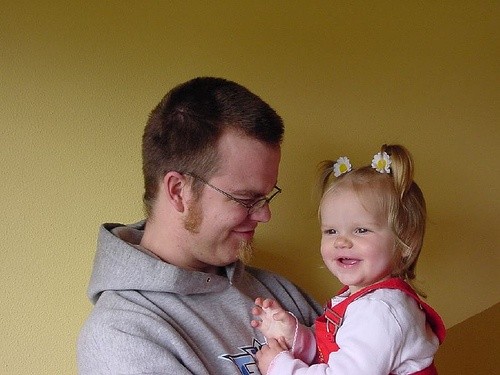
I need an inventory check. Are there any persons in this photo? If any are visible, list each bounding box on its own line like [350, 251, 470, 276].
[250, 142, 447, 375]
[74, 73, 325, 375]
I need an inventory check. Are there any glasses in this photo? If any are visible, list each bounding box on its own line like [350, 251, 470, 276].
[184, 173, 282, 213]
